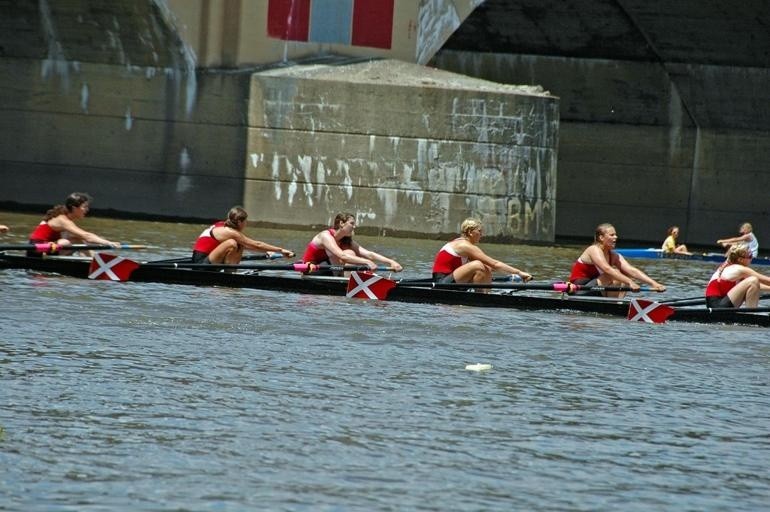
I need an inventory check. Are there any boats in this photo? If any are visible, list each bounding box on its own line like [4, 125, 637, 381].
[0, 253, 769, 326]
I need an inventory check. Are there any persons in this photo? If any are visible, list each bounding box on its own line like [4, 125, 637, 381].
[190, 205, 294, 273]
[0, 224, 9, 233]
[563, 222, 667, 303]
[661, 225, 692, 256]
[430, 215, 534, 293]
[25, 191, 121, 257]
[715, 222, 760, 259]
[300, 210, 404, 279]
[704, 241, 770, 314]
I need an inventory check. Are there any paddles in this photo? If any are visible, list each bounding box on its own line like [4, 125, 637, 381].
[88, 253, 396, 283]
[628, 300, 770, 322]
[347, 271, 666, 299]
